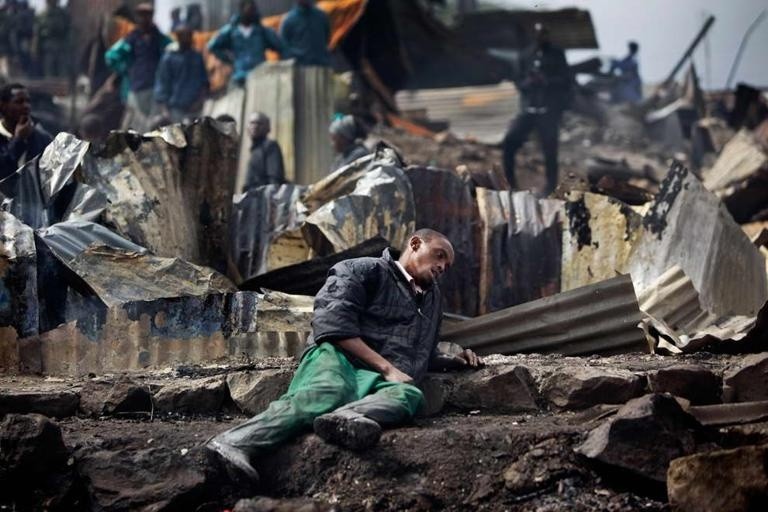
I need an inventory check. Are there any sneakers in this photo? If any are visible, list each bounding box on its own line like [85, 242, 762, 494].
[312, 404, 382, 455]
[202, 435, 260, 490]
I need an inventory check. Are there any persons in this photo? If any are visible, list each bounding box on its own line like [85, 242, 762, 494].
[81, 0, 330, 140]
[611, 40, 643, 102]
[0, 1, 68, 77]
[1, 83, 55, 178]
[500, 16, 583, 198]
[242, 112, 285, 191]
[203, 223, 490, 488]
[327, 114, 367, 173]
